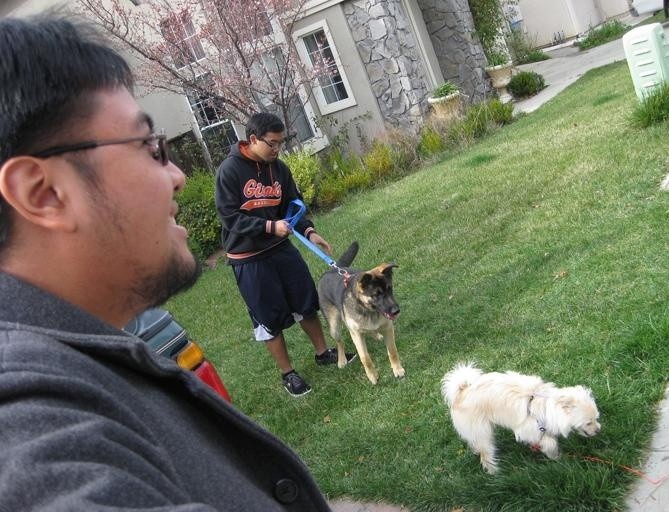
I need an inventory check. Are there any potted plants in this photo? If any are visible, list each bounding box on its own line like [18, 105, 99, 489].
[483, 49, 514, 104]
[426, 78, 464, 119]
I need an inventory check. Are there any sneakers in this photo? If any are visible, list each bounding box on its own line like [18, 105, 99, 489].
[282, 372, 312, 398]
[315, 348, 356, 367]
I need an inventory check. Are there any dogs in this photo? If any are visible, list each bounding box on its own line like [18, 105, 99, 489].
[317, 240, 406, 385]
[439, 361, 602, 477]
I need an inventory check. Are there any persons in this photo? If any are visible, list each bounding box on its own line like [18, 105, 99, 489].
[214, 112, 357, 397]
[0, 12, 337, 512]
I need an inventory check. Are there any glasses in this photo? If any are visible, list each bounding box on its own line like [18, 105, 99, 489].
[259, 136, 286, 150]
[31, 126, 169, 167]
